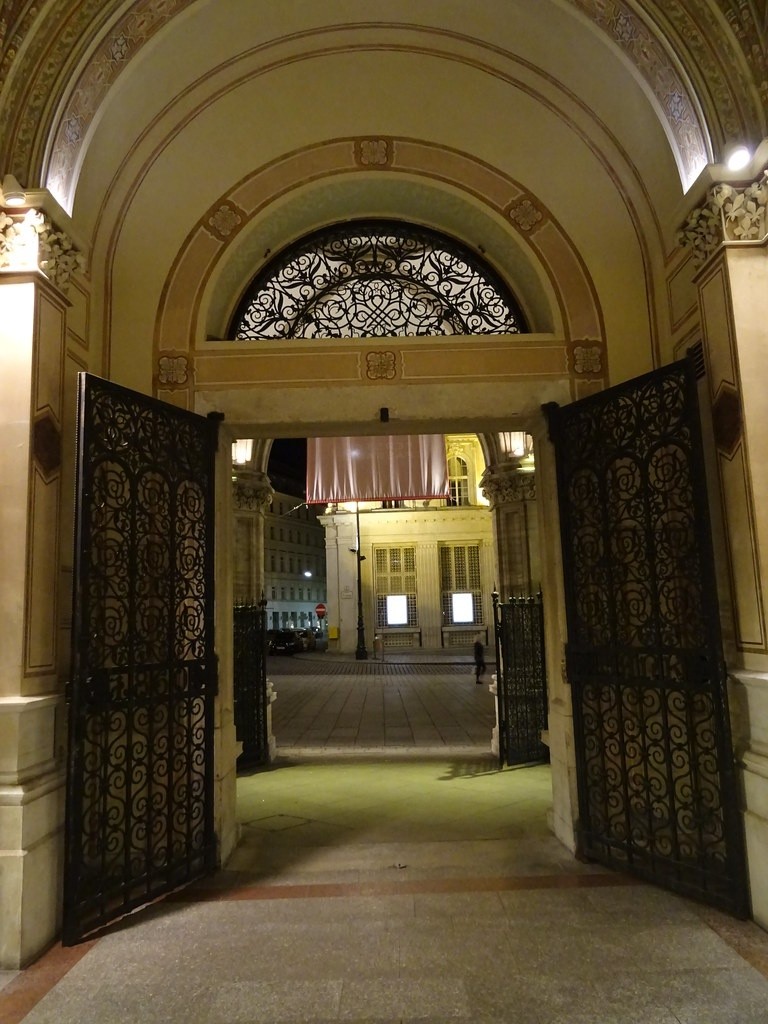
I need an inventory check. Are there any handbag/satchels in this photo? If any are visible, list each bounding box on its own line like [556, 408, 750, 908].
[473, 665, 478, 676]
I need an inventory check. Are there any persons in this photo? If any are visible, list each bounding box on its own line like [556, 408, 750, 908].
[301, 632, 308, 649]
[473, 634, 487, 684]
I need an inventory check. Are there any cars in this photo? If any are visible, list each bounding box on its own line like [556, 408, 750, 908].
[308, 627, 323, 639]
[269, 630, 316, 656]
[268, 626, 294, 646]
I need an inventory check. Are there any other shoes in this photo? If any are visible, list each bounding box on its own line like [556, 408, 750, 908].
[476, 680, 483, 684]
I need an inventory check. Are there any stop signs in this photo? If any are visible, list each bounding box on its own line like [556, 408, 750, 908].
[316, 604, 327, 618]
